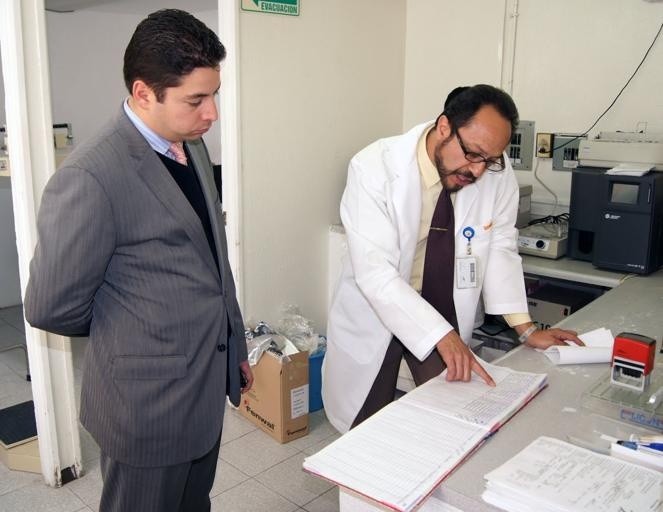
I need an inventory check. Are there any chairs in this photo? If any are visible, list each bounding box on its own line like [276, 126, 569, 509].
[395, 336, 484, 393]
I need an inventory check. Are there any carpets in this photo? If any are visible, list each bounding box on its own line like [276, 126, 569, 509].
[0, 399, 37, 449]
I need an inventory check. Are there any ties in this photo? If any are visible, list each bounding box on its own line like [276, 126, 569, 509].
[167, 140, 190, 168]
[420, 185, 456, 329]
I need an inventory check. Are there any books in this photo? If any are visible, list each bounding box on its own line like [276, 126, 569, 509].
[299, 345, 550, 512]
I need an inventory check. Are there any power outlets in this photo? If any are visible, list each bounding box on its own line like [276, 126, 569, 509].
[536, 133, 554, 158]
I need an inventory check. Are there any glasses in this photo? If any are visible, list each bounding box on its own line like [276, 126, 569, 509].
[449, 120, 507, 173]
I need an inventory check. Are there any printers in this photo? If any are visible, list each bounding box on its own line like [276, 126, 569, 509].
[576, 130, 663, 172]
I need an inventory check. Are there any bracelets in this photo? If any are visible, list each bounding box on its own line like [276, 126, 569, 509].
[517, 323, 537, 343]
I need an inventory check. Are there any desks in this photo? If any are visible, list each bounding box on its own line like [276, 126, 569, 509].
[339, 274, 663, 512]
[328, 225, 638, 364]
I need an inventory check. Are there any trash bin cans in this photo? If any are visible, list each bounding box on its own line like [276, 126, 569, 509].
[309, 335, 327, 412]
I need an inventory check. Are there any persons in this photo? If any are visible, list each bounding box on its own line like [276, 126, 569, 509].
[319, 83, 588, 434]
[22, 6, 258, 511]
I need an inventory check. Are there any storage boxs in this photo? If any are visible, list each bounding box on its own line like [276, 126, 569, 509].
[240, 324, 310, 445]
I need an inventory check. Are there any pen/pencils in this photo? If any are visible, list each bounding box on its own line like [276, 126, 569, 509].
[617, 440, 663, 451]
